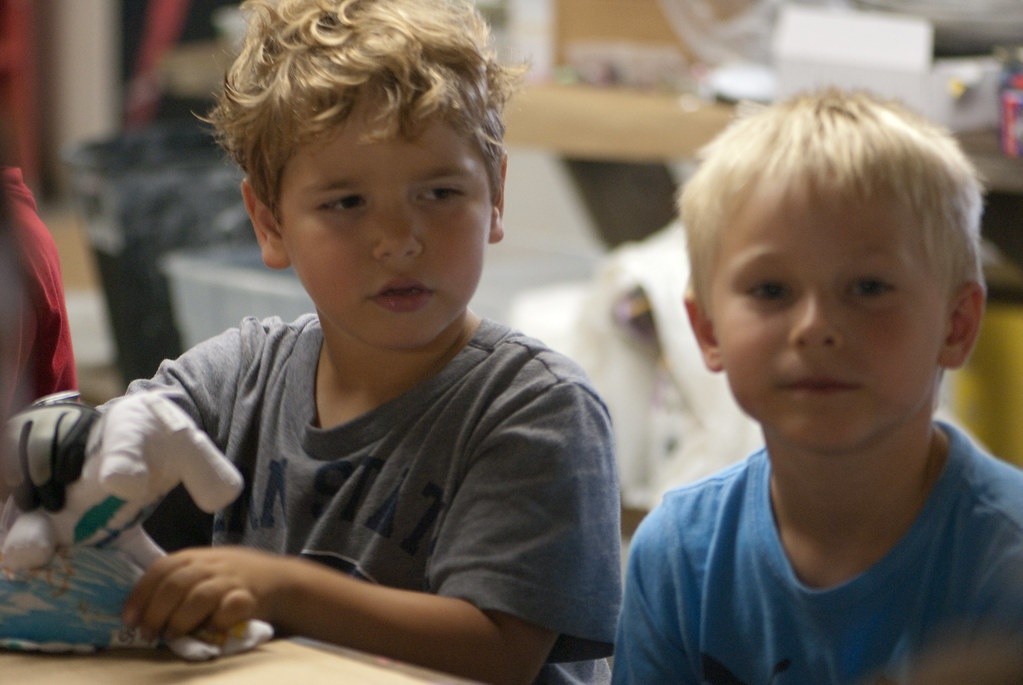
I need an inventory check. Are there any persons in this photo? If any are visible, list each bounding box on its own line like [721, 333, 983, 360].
[611, 84, 1023, 685]
[0, 65, 81, 504]
[3, 0, 625, 685]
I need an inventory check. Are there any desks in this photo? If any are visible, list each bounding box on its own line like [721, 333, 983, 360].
[0, 597, 482, 684]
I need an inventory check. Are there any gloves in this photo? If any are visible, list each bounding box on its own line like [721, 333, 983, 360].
[2, 388, 103, 511]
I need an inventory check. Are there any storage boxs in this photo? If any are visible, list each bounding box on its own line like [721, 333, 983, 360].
[165, 243, 314, 354]
